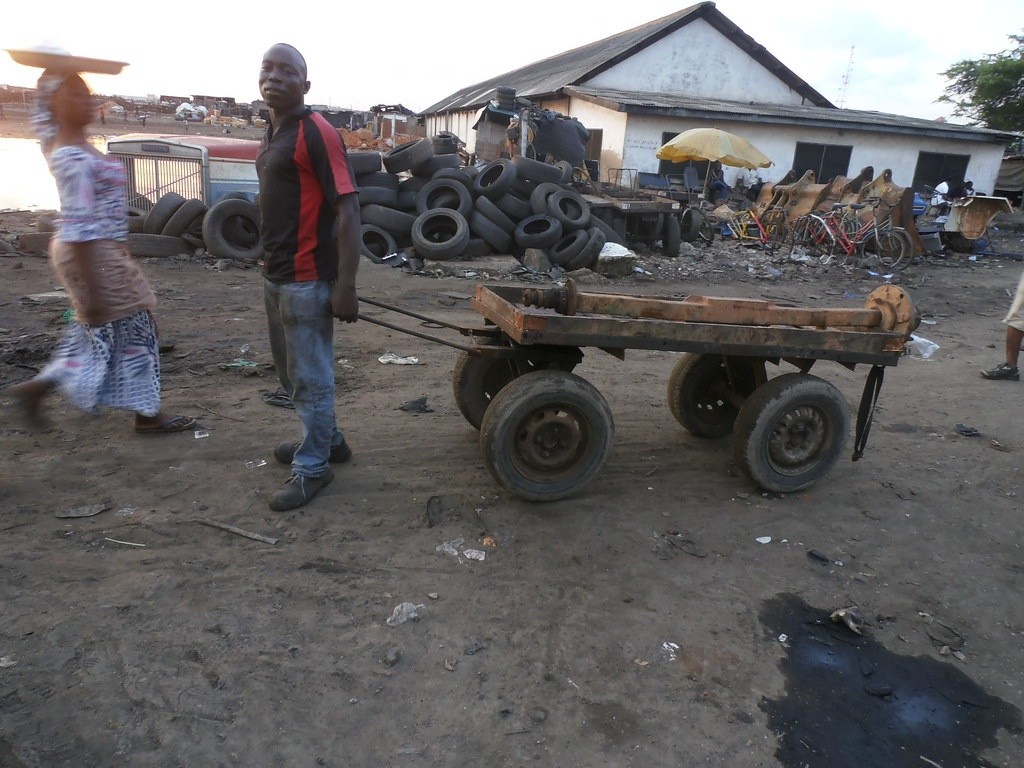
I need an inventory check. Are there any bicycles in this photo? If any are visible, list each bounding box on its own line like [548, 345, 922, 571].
[724, 194, 916, 275]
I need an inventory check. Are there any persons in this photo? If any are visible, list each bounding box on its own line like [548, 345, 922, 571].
[977, 259, 1024, 383]
[121, 107, 129, 125]
[0, 100, 8, 120]
[140, 116, 146, 129]
[248, 40, 364, 516]
[734, 162, 770, 196]
[705, 159, 734, 195]
[6, 43, 199, 438]
[99, 106, 106, 125]
[134, 104, 140, 118]
[182, 116, 189, 130]
[931, 176, 954, 214]
[953, 179, 975, 198]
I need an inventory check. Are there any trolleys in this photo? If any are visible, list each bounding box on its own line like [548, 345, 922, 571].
[356, 279, 912, 507]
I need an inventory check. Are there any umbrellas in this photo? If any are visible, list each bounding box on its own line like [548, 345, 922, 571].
[655, 119, 774, 198]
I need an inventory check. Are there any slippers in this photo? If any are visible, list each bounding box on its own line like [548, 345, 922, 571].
[134, 412, 198, 433]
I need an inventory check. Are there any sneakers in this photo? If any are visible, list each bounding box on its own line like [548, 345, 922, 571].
[980, 360, 1020, 382]
[269, 465, 335, 512]
[273, 438, 353, 467]
